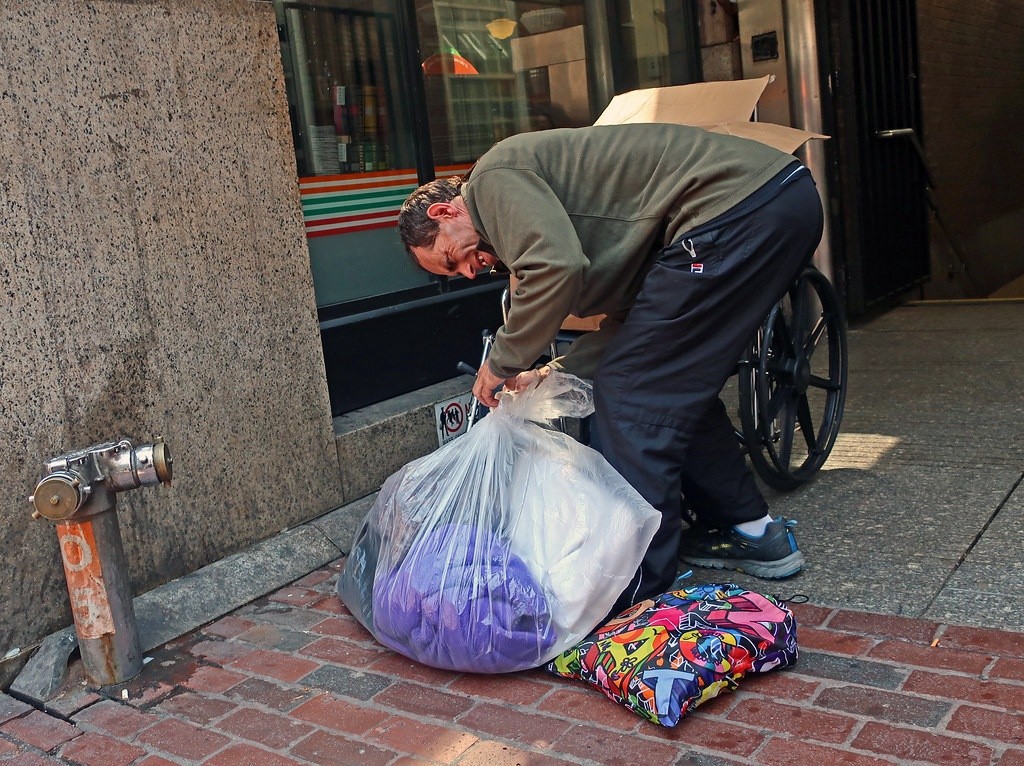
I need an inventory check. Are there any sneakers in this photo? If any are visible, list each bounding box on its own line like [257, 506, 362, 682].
[679, 518, 805, 580]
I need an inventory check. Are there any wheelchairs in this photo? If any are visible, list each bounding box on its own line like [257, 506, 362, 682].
[455, 261, 848, 491]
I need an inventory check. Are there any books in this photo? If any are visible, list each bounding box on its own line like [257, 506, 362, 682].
[296, 4, 410, 170]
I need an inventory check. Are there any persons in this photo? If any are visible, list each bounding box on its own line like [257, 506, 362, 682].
[397, 122, 824, 618]
